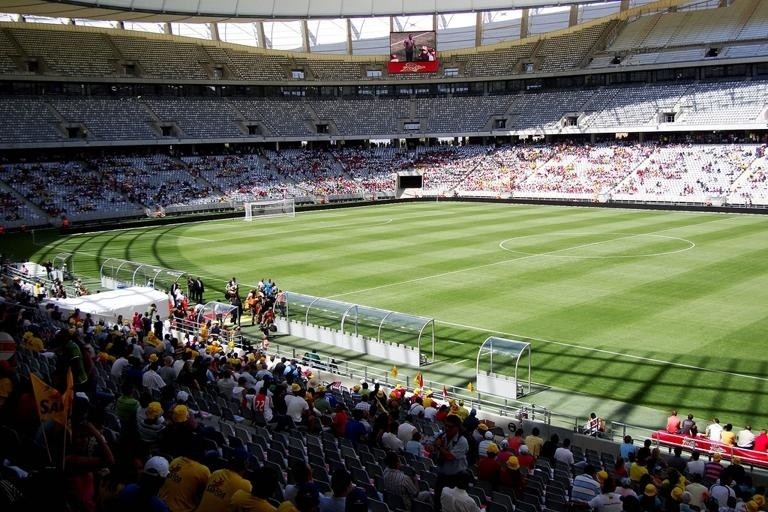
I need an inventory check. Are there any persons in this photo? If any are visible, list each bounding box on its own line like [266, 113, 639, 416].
[0, 255, 288, 341]
[0, 314, 768, 512]
[403, 33, 418, 64]
[478, 138, 768, 208]
[0, 141, 480, 229]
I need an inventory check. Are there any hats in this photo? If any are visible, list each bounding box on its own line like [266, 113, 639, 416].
[595, 470, 609, 483]
[671, 487, 691, 502]
[488, 438, 529, 471]
[145, 402, 165, 420]
[177, 390, 189, 402]
[148, 353, 173, 365]
[144, 456, 171, 479]
[173, 404, 189, 422]
[477, 423, 493, 440]
[74, 391, 90, 410]
[294, 482, 321, 507]
[644, 484, 658, 497]
[746, 495, 765, 510]
[229, 449, 253, 467]
[345, 486, 368, 511]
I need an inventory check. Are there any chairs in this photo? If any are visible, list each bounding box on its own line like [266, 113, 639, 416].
[0, 317, 768, 512]
